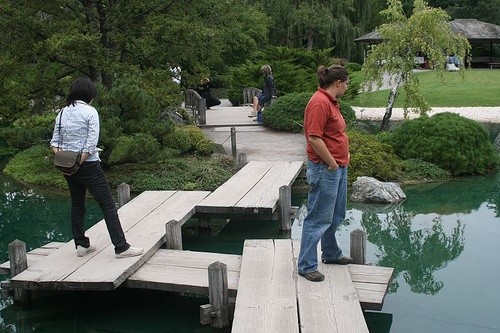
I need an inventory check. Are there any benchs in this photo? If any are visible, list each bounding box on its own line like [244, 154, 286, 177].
[488, 63, 500, 69]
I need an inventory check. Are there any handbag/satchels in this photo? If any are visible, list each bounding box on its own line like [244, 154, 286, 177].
[54, 151, 80, 173]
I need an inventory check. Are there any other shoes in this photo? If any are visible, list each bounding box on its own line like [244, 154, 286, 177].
[253, 118, 258, 121]
[248, 112, 257, 117]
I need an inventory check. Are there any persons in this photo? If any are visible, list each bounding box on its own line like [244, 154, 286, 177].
[195, 79, 221, 109]
[297, 64, 353, 282]
[50, 78, 143, 258]
[248, 64, 277, 121]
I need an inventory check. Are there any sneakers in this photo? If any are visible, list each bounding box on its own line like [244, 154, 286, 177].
[322, 256, 353, 265]
[298, 271, 324, 282]
[116, 247, 144, 259]
[75, 244, 95, 257]
